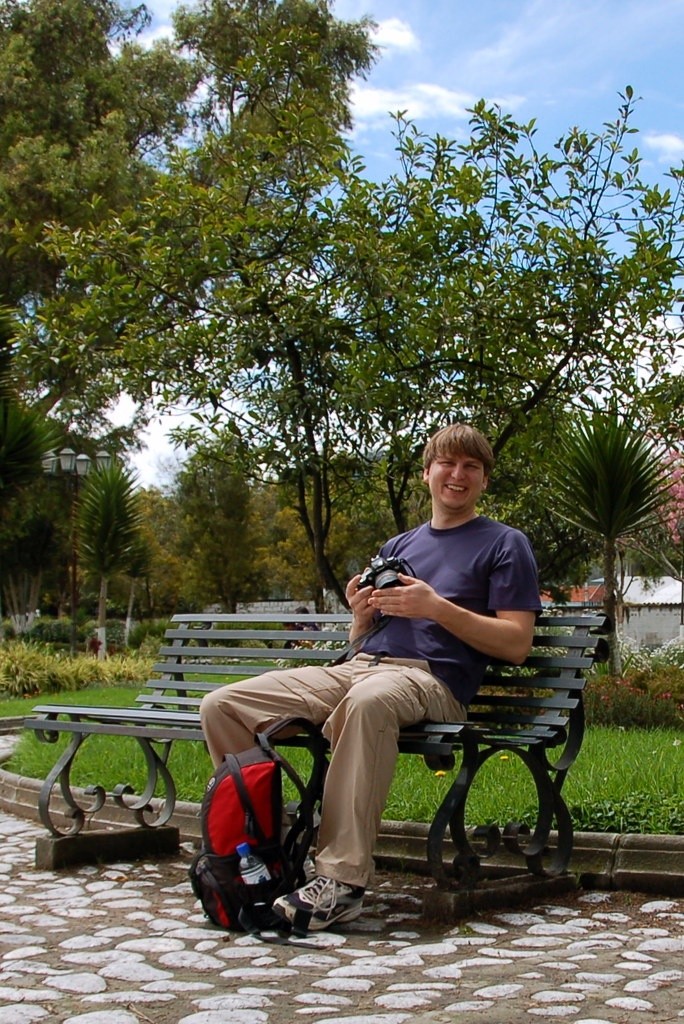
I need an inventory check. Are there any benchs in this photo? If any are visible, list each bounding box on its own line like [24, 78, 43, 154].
[24, 612, 614, 892]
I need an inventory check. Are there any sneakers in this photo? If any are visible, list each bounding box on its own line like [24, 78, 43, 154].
[272, 877, 364, 929]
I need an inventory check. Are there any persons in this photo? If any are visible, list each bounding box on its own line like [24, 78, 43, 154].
[199, 425, 542, 933]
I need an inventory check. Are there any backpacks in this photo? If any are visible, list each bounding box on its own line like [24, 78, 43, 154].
[189, 718, 328, 938]
